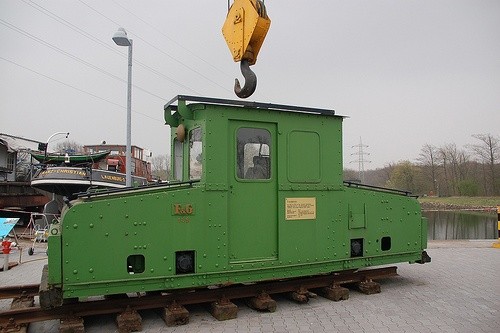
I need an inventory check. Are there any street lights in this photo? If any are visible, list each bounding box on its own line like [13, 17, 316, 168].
[112, 25, 134, 190]
[46, 133, 69, 146]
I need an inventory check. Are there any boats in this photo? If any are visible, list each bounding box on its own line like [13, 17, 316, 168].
[32, 143, 156, 200]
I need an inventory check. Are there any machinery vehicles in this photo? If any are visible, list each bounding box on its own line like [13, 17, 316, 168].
[34, 96, 432, 301]
